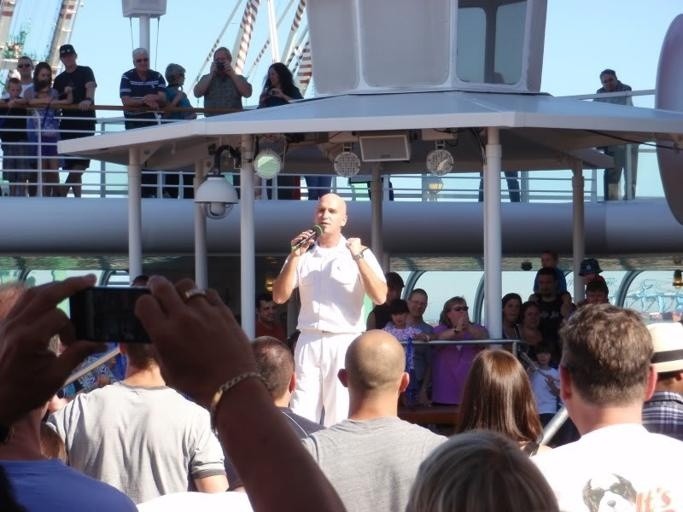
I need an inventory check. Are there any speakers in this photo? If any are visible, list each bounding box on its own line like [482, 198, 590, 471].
[359, 129, 411, 162]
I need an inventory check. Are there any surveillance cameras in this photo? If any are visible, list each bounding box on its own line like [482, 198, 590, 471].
[521, 264, 532, 270]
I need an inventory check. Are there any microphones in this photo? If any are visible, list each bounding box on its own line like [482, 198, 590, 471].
[291, 224, 323, 250]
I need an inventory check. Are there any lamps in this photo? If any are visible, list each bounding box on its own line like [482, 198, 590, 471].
[248, 130, 461, 183]
[192, 144, 245, 222]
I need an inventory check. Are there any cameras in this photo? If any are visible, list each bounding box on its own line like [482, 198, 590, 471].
[216, 63, 224, 69]
[269, 90, 276, 96]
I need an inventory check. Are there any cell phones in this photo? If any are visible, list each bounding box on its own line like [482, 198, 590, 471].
[70, 286, 154, 342]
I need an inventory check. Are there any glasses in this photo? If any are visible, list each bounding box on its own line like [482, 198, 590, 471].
[602, 77, 616, 84]
[16, 63, 33, 69]
[448, 306, 469, 312]
[134, 58, 150, 63]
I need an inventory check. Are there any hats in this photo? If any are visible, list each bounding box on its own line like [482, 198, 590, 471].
[642, 320, 683, 376]
[577, 258, 604, 277]
[59, 44, 75, 59]
